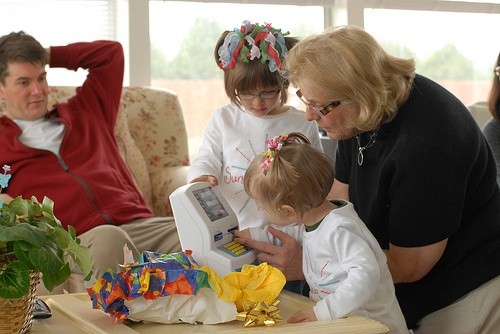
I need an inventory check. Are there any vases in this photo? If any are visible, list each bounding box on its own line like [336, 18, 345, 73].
[0, 267, 39, 334]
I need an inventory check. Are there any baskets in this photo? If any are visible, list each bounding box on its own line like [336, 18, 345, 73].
[0, 267, 40, 334]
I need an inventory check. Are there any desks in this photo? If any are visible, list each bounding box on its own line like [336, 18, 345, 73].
[31, 290, 389, 334]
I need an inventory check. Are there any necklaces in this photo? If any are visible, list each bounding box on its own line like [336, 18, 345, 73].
[356, 129, 377, 166]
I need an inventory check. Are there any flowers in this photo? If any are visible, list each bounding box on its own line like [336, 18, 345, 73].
[0, 163, 93, 298]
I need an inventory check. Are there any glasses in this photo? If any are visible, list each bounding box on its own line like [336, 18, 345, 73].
[235, 87, 280, 99]
[296, 89, 351, 118]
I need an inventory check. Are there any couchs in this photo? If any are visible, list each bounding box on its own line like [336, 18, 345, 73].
[0, 86, 192, 216]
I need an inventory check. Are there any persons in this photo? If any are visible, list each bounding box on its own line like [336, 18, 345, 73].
[482, 53, 500, 187]
[232, 132, 409, 334]
[233, 24, 500, 334]
[189, 20, 339, 231]
[0, 32, 182, 291]
